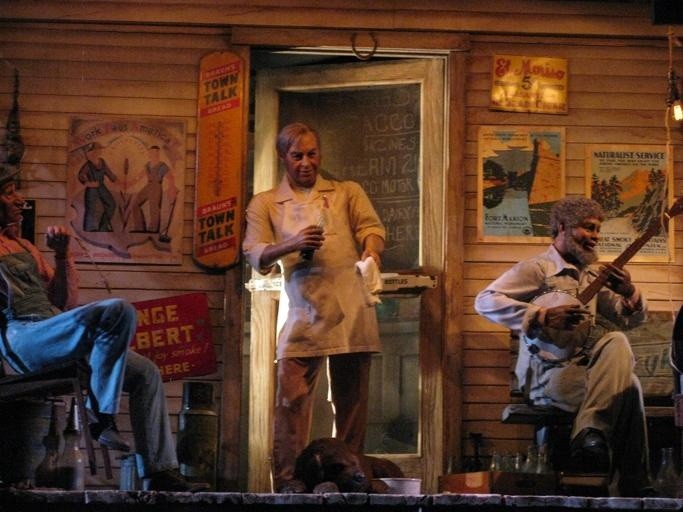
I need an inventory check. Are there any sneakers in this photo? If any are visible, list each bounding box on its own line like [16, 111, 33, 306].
[143, 468, 212, 491]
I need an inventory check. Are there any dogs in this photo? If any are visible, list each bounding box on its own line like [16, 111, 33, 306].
[278, 438, 406, 494]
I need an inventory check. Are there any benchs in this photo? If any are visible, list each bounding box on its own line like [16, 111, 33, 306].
[501, 309, 683, 477]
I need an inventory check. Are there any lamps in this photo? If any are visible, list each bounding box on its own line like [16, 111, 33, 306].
[665, 69, 683, 122]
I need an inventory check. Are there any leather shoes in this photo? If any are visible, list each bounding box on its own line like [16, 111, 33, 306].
[83, 407, 131, 452]
[571, 428, 609, 466]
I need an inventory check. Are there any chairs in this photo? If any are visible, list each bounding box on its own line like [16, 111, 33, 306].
[0, 371, 115, 477]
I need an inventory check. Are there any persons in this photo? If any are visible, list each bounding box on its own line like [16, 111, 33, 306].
[80, 141, 123, 234]
[239, 120, 389, 496]
[123, 146, 178, 234]
[473, 195, 660, 497]
[1, 162, 210, 492]
